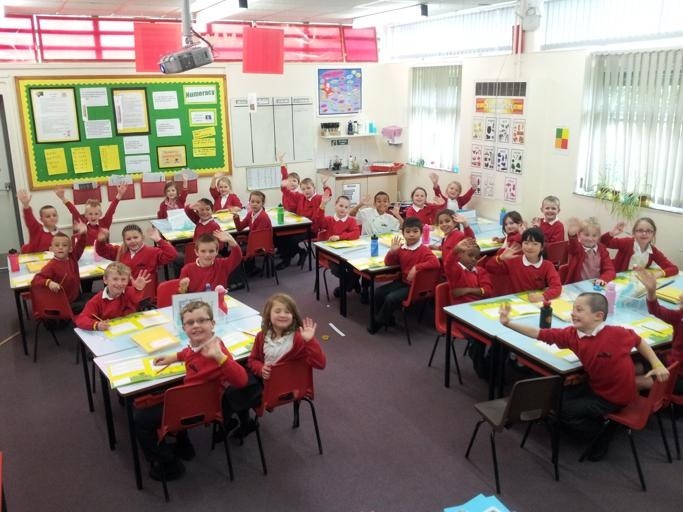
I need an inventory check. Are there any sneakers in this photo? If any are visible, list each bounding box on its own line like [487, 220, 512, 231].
[229, 420, 259, 441]
[150, 441, 195, 480]
[333, 272, 369, 304]
[276, 260, 290, 270]
[297, 248, 307, 265]
[216, 418, 238, 442]
[368, 316, 395, 334]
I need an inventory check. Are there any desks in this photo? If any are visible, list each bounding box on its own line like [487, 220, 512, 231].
[93, 314, 268, 490]
[616, 262, 682, 304]
[443, 277, 645, 406]
[73, 292, 261, 414]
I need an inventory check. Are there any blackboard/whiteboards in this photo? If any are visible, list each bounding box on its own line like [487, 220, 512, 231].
[230, 96, 315, 168]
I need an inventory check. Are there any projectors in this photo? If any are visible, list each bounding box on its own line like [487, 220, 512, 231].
[159, 45, 213, 74]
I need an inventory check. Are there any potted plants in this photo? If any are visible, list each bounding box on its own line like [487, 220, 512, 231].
[594, 170, 652, 225]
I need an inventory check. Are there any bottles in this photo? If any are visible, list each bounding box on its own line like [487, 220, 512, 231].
[214, 284, 228, 314]
[348, 154, 353, 169]
[538, 297, 553, 330]
[421, 223, 429, 245]
[593, 285, 601, 294]
[370, 234, 378, 256]
[276, 203, 283, 224]
[93, 240, 102, 262]
[499, 207, 506, 225]
[604, 281, 615, 312]
[347, 120, 361, 136]
[7, 248, 20, 272]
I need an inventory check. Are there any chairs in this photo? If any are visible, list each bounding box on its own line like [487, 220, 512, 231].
[425, 274, 494, 381]
[574, 362, 682, 491]
[138, 372, 236, 501]
[7, 204, 562, 362]
[211, 352, 324, 475]
[464, 373, 564, 495]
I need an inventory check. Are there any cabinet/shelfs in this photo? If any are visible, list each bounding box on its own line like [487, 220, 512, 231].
[316, 161, 398, 218]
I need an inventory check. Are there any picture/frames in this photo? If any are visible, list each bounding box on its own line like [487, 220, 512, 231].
[317, 68, 363, 115]
[28, 86, 81, 143]
[111, 87, 150, 136]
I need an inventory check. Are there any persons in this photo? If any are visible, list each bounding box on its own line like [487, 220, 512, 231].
[564, 215, 617, 284]
[486, 195, 562, 301]
[18, 189, 58, 254]
[221, 291, 326, 438]
[443, 236, 508, 383]
[134, 300, 249, 482]
[276, 151, 332, 271]
[157, 174, 273, 289]
[600, 218, 677, 278]
[30, 217, 178, 347]
[54, 183, 128, 246]
[312, 172, 479, 334]
[633, 263, 683, 396]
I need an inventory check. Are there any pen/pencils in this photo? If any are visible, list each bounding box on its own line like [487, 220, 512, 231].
[642, 325, 664, 334]
[320, 122, 339, 132]
[157, 364, 170, 374]
[242, 332, 256, 337]
[59, 273, 67, 287]
[91, 313, 112, 326]
